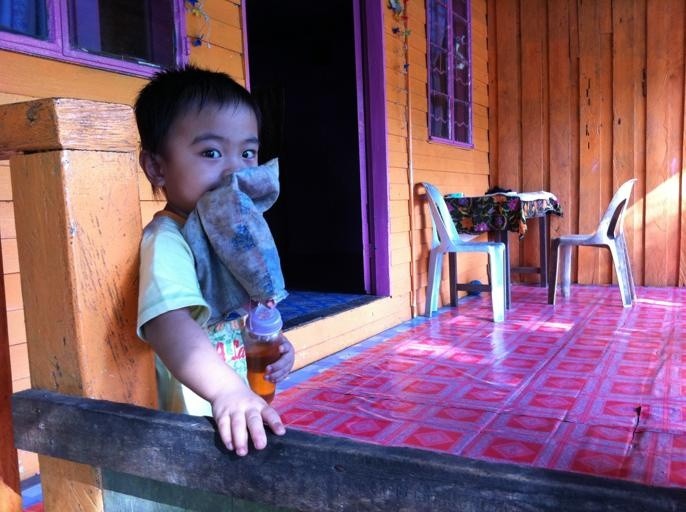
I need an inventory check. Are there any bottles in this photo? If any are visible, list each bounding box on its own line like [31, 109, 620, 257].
[242, 296, 283, 404]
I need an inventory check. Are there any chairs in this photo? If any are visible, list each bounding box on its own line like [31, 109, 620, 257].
[547, 178, 639, 308]
[421, 181, 506, 321]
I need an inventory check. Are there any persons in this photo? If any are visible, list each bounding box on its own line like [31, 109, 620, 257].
[133, 61, 296, 458]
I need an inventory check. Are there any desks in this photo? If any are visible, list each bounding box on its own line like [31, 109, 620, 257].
[440, 190, 564, 308]
